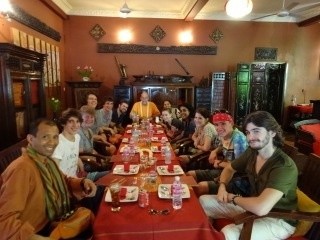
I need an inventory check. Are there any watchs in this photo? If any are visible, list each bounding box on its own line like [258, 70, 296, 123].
[80, 178, 85, 189]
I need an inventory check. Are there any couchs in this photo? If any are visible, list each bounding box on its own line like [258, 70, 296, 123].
[293, 120, 320, 156]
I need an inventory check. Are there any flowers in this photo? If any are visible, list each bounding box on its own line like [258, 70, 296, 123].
[75, 64, 93, 78]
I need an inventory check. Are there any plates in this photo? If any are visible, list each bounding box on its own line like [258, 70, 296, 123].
[152, 146, 166, 152]
[127, 130, 132, 134]
[155, 165, 185, 175]
[156, 124, 162, 128]
[151, 137, 168, 141]
[298, 103, 309, 106]
[120, 147, 139, 153]
[122, 137, 129, 143]
[105, 186, 139, 203]
[127, 124, 132, 127]
[157, 130, 164, 133]
[113, 165, 139, 175]
[158, 184, 191, 199]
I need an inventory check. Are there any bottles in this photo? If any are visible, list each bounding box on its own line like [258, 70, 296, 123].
[130, 145, 135, 164]
[148, 157, 157, 179]
[139, 155, 148, 179]
[139, 188, 145, 207]
[123, 145, 129, 163]
[172, 176, 182, 210]
[165, 142, 172, 164]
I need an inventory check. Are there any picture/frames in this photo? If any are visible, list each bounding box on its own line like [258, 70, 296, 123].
[253, 46, 278, 62]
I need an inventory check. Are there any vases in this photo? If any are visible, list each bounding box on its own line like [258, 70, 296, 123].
[82, 77, 89, 82]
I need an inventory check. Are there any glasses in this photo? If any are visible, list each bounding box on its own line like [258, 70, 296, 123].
[213, 109, 232, 115]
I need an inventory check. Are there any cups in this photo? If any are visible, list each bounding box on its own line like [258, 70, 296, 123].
[168, 163, 173, 171]
[158, 143, 161, 151]
[291, 96, 296, 106]
[124, 161, 129, 170]
[109, 182, 121, 212]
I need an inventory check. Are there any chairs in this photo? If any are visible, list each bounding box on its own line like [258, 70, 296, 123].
[176, 136, 191, 145]
[215, 143, 320, 240]
[79, 139, 110, 163]
[97, 127, 113, 142]
[196, 153, 216, 169]
[81, 158, 101, 172]
[0, 138, 26, 174]
[180, 140, 210, 162]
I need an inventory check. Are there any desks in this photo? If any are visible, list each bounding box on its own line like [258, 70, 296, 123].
[287, 103, 314, 121]
[309, 99, 320, 119]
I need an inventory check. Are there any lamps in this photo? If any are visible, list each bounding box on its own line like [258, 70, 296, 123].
[1, 1, 15, 23]
[224, 0, 253, 19]
[118, 0, 131, 18]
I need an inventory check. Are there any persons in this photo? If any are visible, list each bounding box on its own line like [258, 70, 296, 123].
[52, 108, 109, 195]
[95, 98, 122, 143]
[187, 110, 250, 193]
[168, 103, 196, 143]
[0, 118, 94, 239]
[79, 105, 113, 170]
[175, 106, 220, 167]
[199, 111, 299, 240]
[111, 101, 129, 127]
[129, 91, 161, 121]
[83, 92, 120, 154]
[160, 100, 182, 128]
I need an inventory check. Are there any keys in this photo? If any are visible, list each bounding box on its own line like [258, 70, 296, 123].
[148, 207, 170, 216]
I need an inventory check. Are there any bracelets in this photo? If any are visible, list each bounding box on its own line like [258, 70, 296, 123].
[219, 182, 226, 186]
[217, 160, 223, 168]
[231, 194, 242, 206]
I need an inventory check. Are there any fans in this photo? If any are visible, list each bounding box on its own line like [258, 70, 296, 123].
[250, 0, 305, 23]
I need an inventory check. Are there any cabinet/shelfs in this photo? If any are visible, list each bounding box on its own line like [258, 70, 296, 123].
[209, 72, 230, 114]
[234, 63, 251, 126]
[195, 86, 210, 118]
[250, 63, 265, 113]
[264, 62, 289, 123]
[132, 82, 194, 116]
[112, 85, 133, 124]
[65, 80, 104, 109]
[0, 42, 49, 151]
